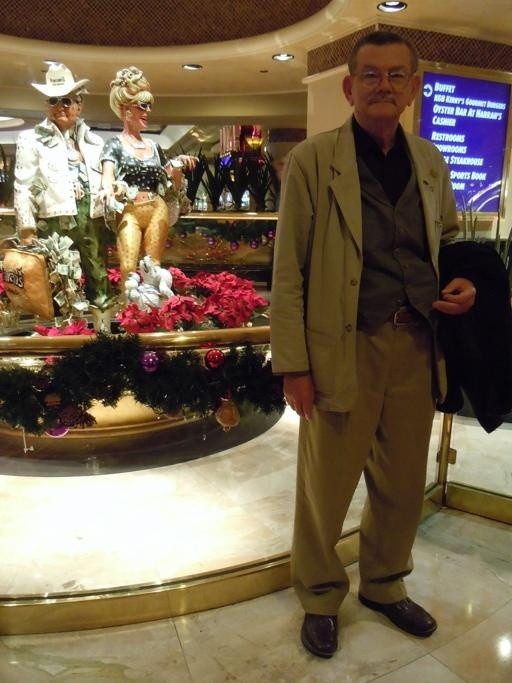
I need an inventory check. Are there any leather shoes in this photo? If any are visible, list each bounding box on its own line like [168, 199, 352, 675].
[301, 613, 337, 658]
[359, 592, 437, 636]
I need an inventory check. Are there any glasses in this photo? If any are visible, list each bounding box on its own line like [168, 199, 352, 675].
[49, 97, 72, 108]
[133, 103, 152, 112]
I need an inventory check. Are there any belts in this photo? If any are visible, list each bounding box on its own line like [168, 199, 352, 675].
[386, 310, 424, 326]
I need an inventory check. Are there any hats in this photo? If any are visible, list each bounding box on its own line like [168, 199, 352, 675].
[31, 62, 90, 97]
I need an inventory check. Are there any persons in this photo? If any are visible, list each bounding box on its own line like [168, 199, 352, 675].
[100, 68, 197, 295]
[15, 64, 104, 307]
[269, 31, 478, 659]
